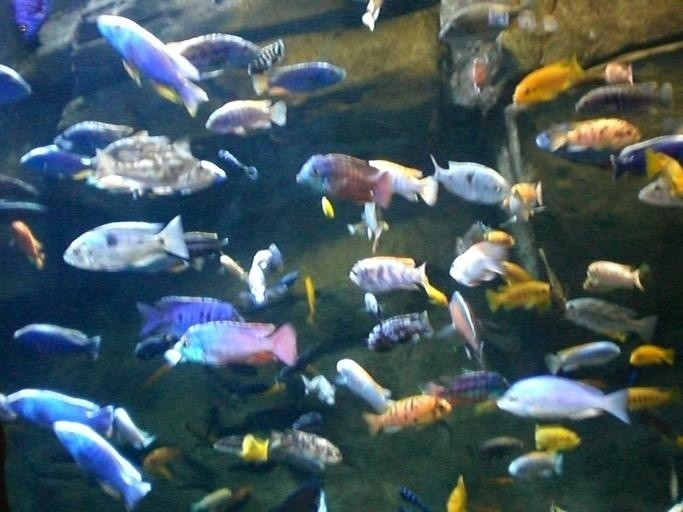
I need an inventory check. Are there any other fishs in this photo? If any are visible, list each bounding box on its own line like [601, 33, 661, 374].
[0, 1, 682, 511]
